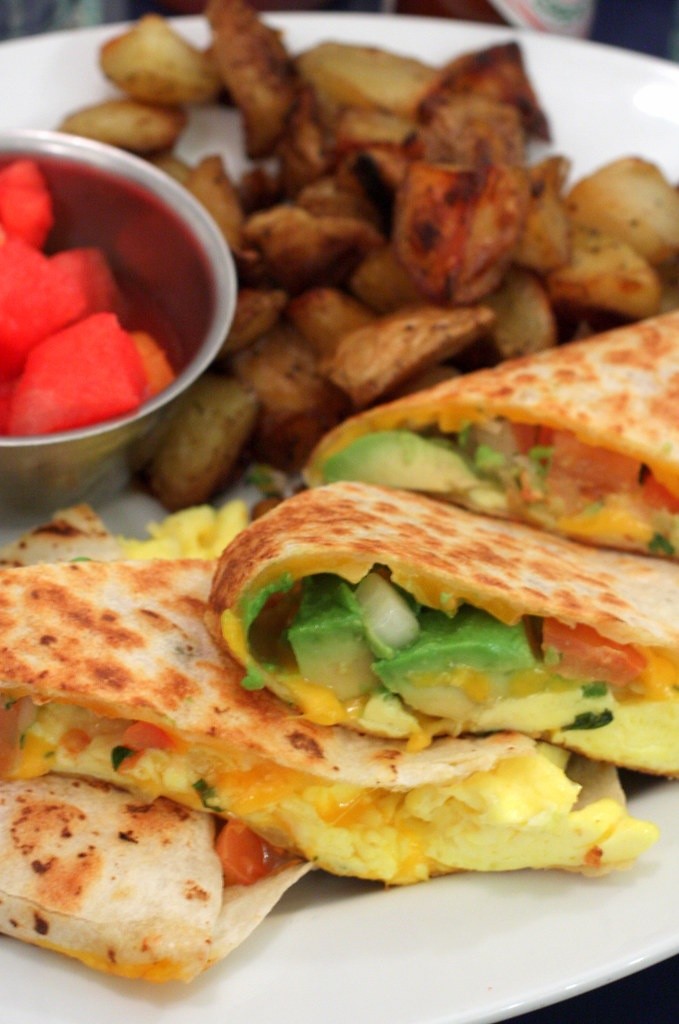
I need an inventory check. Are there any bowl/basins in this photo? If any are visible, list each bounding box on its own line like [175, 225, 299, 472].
[0, 127, 238, 526]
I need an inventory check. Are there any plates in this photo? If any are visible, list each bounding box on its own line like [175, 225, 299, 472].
[0, 13, 679, 1024]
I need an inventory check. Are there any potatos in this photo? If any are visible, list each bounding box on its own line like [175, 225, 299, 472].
[57, 0, 679, 514]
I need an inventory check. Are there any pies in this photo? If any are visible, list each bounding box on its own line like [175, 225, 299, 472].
[0, 308, 679, 987]
